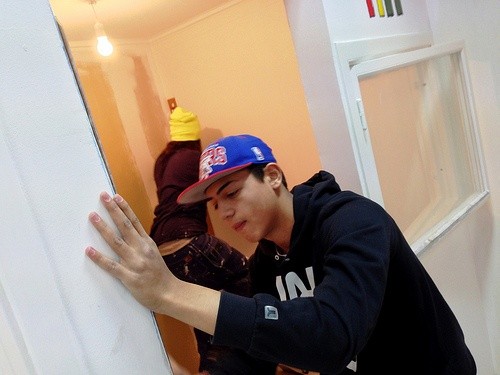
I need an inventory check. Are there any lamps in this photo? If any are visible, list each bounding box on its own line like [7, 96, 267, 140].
[93, 22, 114, 57]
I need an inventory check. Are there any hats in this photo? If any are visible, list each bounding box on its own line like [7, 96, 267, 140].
[177, 135, 277, 204]
[169, 107, 201, 141]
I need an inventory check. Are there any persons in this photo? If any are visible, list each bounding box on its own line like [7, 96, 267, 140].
[86, 135, 477, 375]
[149, 107, 249, 374]
